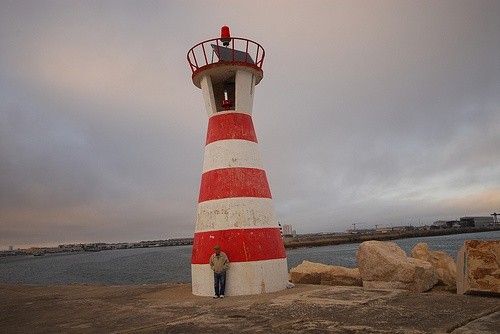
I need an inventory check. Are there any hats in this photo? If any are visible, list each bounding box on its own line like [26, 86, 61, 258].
[214, 245, 221, 249]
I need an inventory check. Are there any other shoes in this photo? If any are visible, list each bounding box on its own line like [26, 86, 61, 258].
[219, 295, 224, 298]
[213, 295, 218, 299]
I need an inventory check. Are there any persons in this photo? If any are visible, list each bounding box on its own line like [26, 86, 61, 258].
[209, 245, 229, 299]
